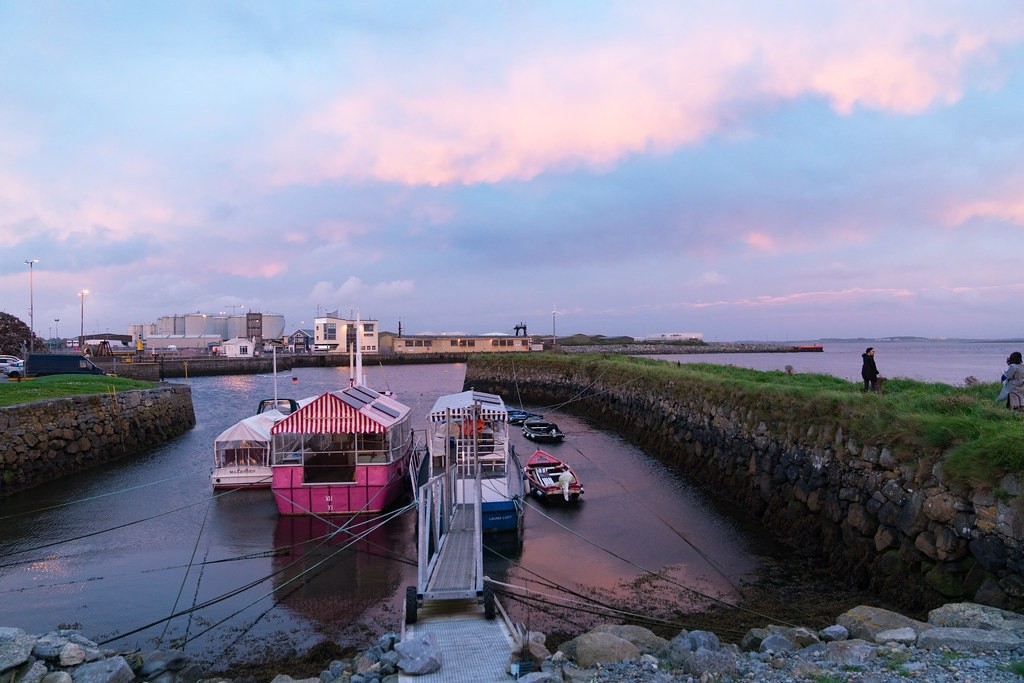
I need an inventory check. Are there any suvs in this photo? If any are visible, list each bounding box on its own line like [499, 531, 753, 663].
[0, 355, 21, 373]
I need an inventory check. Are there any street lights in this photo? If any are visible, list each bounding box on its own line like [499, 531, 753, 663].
[78, 290, 89, 348]
[23, 259, 38, 353]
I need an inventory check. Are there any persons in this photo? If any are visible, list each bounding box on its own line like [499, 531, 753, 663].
[460, 417, 486, 444]
[996, 351, 1024, 412]
[861, 347, 879, 393]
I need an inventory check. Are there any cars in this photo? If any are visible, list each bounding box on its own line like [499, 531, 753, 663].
[2, 360, 26, 378]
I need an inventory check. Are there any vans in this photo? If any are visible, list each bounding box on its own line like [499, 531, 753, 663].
[27, 353, 104, 378]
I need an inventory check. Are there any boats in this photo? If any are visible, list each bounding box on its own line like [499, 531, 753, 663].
[521, 416, 566, 444]
[504, 405, 545, 426]
[409, 388, 526, 546]
[208, 393, 324, 488]
[269, 312, 418, 517]
[524, 446, 584, 506]
